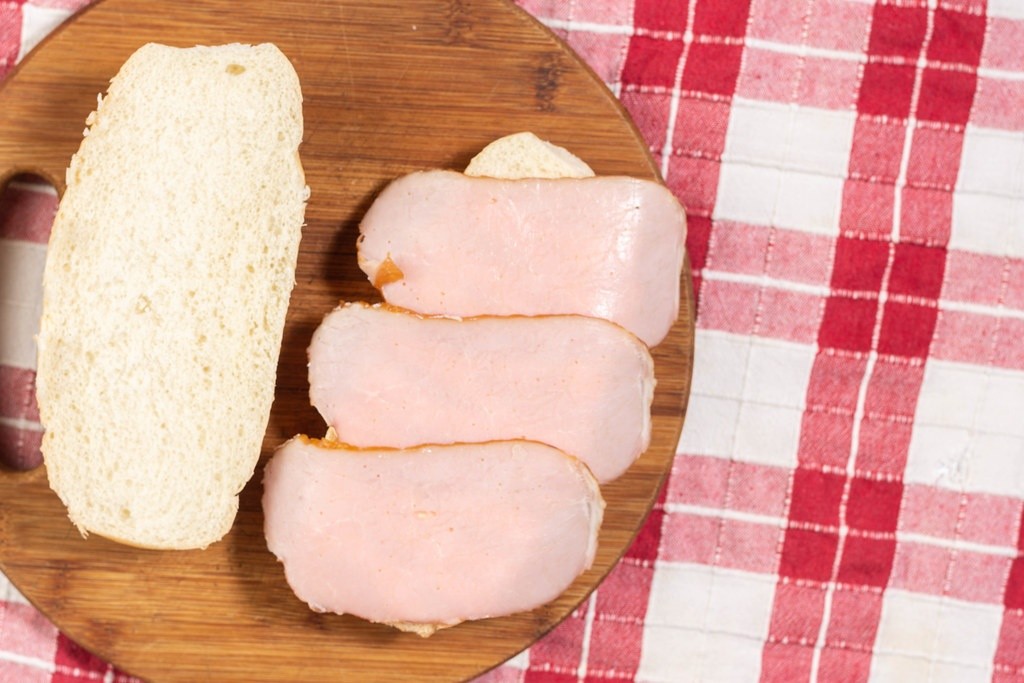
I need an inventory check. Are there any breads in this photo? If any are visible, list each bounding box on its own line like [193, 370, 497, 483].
[325, 133, 595, 637]
[38, 42, 311, 551]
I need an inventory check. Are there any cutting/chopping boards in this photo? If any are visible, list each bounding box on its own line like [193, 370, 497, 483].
[0, 1, 694, 683]
